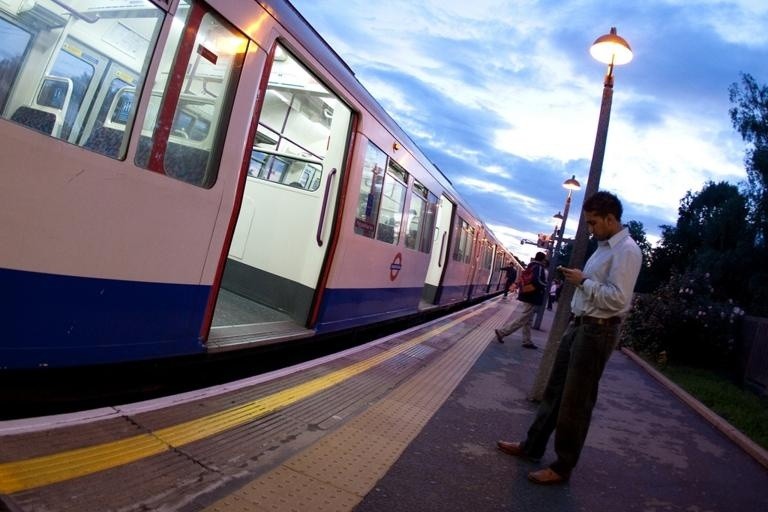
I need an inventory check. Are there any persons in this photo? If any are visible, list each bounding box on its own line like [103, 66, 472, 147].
[499, 261, 517, 300]
[530, 257, 551, 330]
[546, 279, 558, 311]
[492, 190, 644, 485]
[494, 251, 548, 349]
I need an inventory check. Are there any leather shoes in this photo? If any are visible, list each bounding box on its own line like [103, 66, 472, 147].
[527, 467, 564, 484]
[496, 439, 541, 463]
[495, 329, 504, 343]
[521, 343, 538, 349]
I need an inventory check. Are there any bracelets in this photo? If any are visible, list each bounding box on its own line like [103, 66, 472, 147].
[578, 276, 590, 289]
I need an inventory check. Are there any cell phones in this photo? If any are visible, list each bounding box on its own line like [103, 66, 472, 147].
[556, 266, 568, 274]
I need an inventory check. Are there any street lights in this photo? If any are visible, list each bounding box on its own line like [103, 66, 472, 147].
[530, 27, 634, 406]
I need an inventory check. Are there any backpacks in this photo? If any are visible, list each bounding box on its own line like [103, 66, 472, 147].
[517, 263, 538, 293]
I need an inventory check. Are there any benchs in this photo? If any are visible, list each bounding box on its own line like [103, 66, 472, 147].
[9, 105, 209, 189]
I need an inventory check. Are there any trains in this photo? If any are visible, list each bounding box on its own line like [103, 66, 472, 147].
[0, 0, 526, 370]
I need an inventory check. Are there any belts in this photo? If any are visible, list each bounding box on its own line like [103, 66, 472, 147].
[568, 313, 621, 326]
